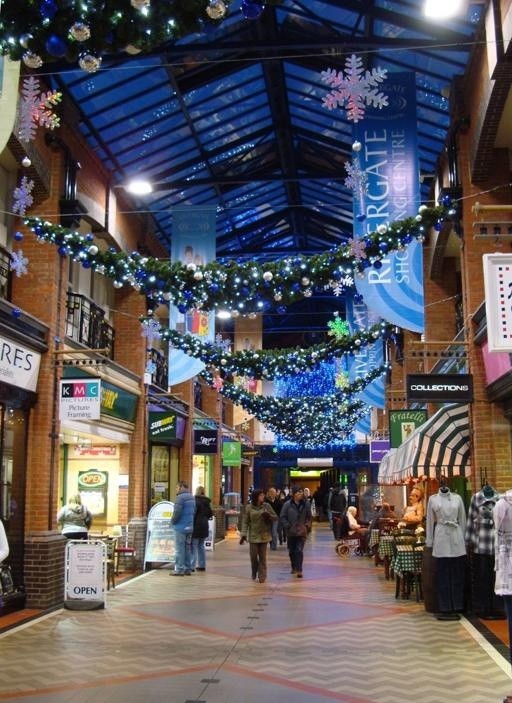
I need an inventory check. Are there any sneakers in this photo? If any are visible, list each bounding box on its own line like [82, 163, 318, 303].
[292, 569, 303, 578]
[169, 567, 205, 575]
[252, 571, 265, 582]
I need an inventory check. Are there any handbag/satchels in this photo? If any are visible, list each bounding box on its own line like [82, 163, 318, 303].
[84, 516, 91, 525]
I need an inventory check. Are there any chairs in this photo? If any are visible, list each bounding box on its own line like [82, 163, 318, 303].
[378, 521, 426, 603]
[89, 535, 109, 544]
[100, 539, 116, 591]
[114, 531, 137, 576]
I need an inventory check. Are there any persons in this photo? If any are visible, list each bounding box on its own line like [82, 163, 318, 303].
[190, 485, 212, 572]
[493, 487, 512, 662]
[0, 520, 10, 562]
[401, 487, 425, 529]
[169, 480, 196, 577]
[57, 492, 92, 544]
[425, 489, 465, 613]
[238, 483, 347, 583]
[182, 245, 193, 266]
[193, 254, 201, 265]
[367, 502, 398, 539]
[345, 506, 369, 552]
[467, 484, 499, 618]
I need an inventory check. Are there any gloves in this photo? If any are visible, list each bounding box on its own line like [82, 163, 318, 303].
[240, 536, 247, 544]
[260, 512, 271, 520]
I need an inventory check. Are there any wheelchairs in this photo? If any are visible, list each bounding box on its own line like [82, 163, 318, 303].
[332, 513, 373, 557]
